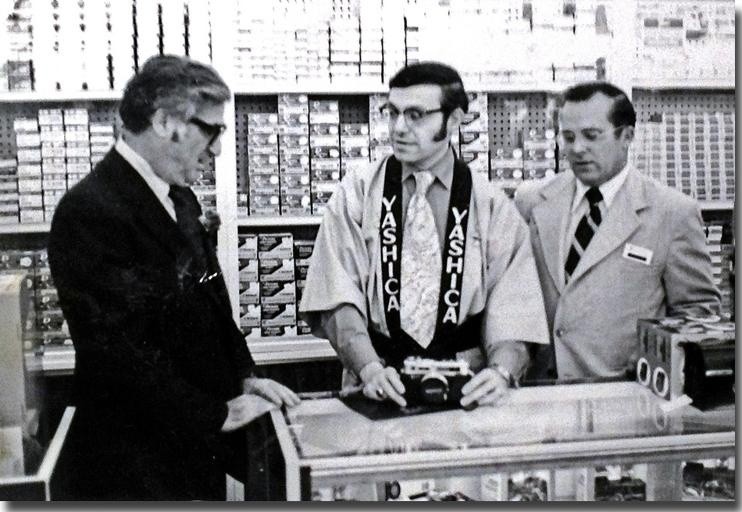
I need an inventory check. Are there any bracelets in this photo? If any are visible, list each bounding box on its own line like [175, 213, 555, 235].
[359, 362, 382, 383]
[484, 358, 515, 386]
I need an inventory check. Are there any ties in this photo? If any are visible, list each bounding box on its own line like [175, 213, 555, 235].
[564, 187, 603, 281]
[400, 169, 447, 350]
[169, 184, 211, 281]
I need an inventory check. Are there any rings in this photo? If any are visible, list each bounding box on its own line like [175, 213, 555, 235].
[377, 387, 384, 392]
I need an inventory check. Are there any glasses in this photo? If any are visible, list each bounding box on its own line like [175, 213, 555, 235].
[378, 102, 444, 127]
[554, 125, 625, 147]
[191, 116, 227, 147]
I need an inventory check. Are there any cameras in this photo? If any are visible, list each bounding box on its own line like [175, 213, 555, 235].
[399, 359, 470, 410]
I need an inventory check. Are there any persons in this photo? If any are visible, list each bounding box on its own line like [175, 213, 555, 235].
[46, 54, 302, 501]
[296, 61, 551, 420]
[510, 83, 724, 385]
